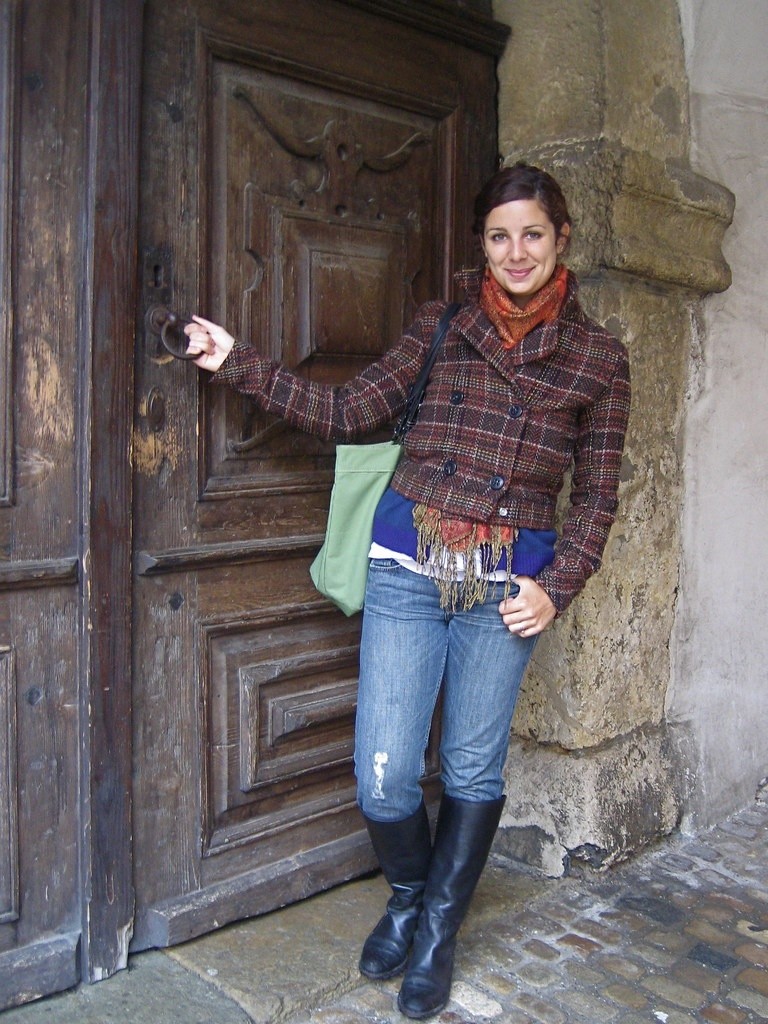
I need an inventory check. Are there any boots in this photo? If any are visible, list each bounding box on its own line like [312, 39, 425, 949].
[398, 789, 506, 1018]
[358, 792, 432, 978]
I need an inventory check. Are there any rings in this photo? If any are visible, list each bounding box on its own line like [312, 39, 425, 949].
[522, 630, 525, 633]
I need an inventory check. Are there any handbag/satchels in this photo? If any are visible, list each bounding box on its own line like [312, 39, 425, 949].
[309, 437, 405, 617]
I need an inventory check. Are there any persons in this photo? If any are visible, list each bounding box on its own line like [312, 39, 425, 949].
[185, 161, 630, 1020]
